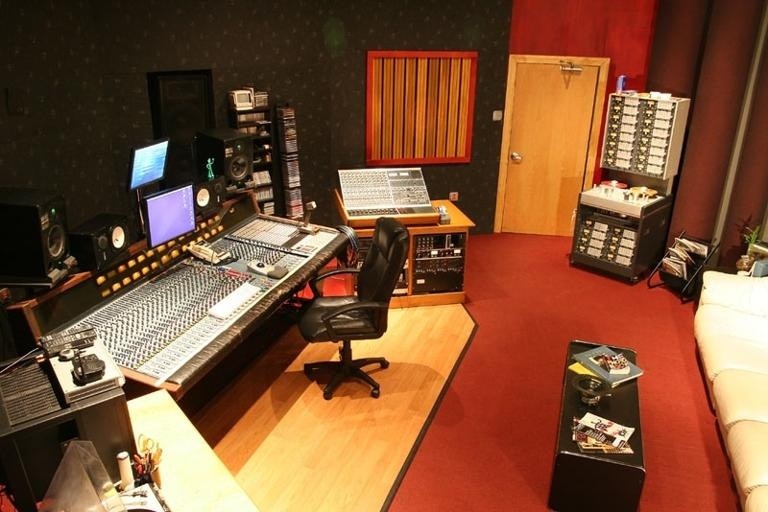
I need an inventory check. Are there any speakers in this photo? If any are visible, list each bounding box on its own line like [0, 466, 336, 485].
[68, 210, 132, 270]
[0, 185, 76, 281]
[193, 175, 229, 221]
[194, 127, 253, 189]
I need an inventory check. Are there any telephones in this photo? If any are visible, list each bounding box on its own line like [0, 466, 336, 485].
[187, 239, 232, 265]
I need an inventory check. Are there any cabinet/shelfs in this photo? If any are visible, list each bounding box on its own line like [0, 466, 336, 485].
[646, 230, 721, 303]
[600, 93, 691, 180]
[229, 104, 304, 221]
[347, 199, 476, 309]
[569, 186, 672, 285]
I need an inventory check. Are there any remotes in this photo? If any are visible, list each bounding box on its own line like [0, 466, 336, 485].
[44, 324, 93, 342]
[42, 330, 98, 349]
[47, 338, 95, 357]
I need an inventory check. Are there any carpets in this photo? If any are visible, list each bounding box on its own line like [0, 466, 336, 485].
[380, 231, 743, 511]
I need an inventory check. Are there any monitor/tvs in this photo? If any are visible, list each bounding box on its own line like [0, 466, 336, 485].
[143, 181, 198, 248]
[127, 135, 171, 192]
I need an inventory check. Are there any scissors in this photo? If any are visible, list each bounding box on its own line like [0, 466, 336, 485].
[138, 434, 154, 455]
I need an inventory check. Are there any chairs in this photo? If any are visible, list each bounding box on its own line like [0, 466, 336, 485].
[295, 217, 409, 398]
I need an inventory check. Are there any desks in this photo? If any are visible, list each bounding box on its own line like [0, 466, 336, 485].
[42, 212, 349, 402]
[127, 389, 259, 512]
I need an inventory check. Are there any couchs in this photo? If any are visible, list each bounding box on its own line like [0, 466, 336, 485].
[694, 271, 767, 511]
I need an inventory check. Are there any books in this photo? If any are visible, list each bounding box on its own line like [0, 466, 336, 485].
[662, 237, 708, 280]
[568, 346, 644, 388]
[571, 412, 636, 455]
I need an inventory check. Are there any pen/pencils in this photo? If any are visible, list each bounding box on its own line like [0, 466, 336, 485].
[134, 452, 154, 482]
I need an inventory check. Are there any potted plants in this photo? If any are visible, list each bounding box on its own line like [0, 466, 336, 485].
[735, 224, 761, 271]
